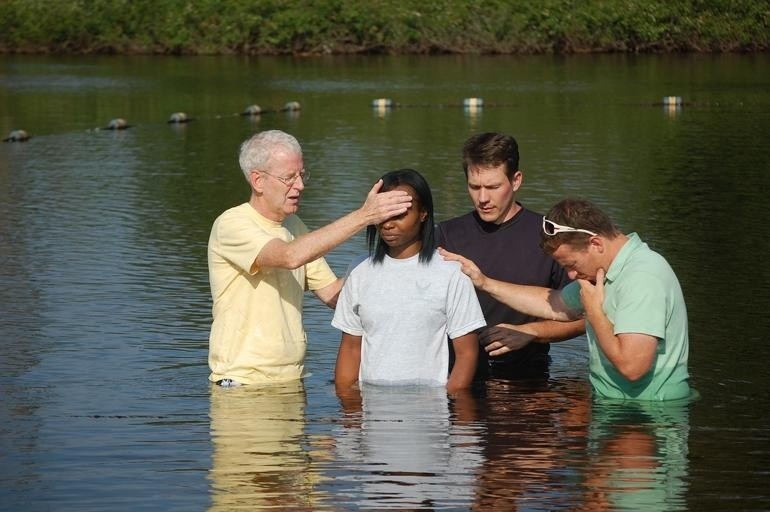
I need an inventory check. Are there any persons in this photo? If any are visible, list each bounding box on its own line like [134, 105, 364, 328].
[438, 197, 690, 399]
[206, 130, 412, 387]
[433, 131, 587, 380]
[335, 168, 486, 397]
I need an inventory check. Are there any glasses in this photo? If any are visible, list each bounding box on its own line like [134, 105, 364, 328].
[257, 170, 311, 184]
[543, 215, 596, 238]
